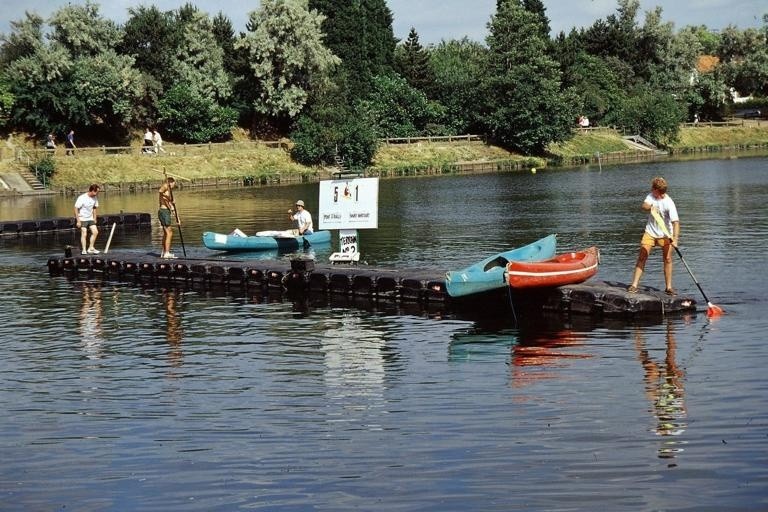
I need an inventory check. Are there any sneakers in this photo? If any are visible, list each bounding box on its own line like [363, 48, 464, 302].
[628, 284, 640, 293]
[82, 249, 88, 255]
[665, 288, 674, 295]
[88, 248, 100, 254]
[161, 251, 174, 259]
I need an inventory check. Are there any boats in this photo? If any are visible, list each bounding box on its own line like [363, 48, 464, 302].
[442, 232, 560, 302]
[199, 230, 333, 252]
[503, 245, 602, 290]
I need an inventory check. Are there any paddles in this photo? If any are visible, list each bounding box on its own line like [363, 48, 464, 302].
[650, 206, 724, 317]
[289, 212, 311, 251]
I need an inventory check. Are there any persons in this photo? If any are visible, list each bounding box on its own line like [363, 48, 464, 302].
[65, 129, 76, 156]
[289, 199, 315, 235]
[145, 128, 166, 156]
[628, 177, 680, 297]
[633, 316, 685, 468]
[158, 179, 178, 259]
[694, 110, 700, 124]
[74, 185, 99, 255]
[47, 132, 57, 157]
[578, 115, 590, 133]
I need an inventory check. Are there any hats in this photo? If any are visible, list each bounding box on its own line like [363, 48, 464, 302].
[296, 200, 305, 207]
